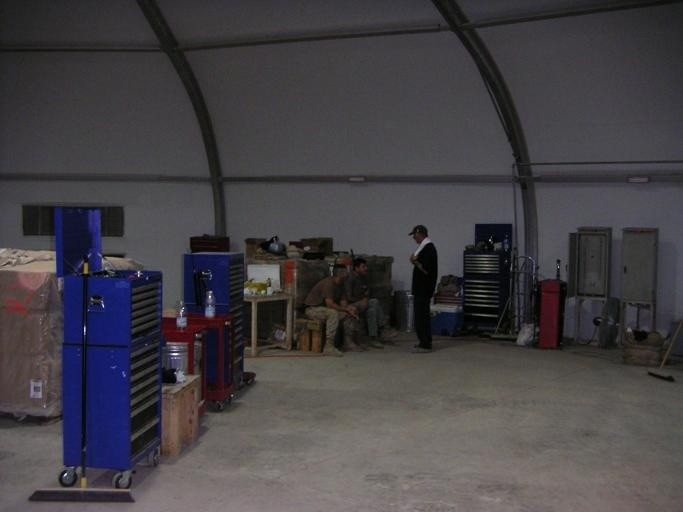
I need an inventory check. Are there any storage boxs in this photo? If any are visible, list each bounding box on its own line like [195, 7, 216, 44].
[162, 374, 201, 459]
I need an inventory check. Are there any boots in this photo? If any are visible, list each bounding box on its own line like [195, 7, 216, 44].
[342, 336, 364, 352]
[323, 340, 344, 357]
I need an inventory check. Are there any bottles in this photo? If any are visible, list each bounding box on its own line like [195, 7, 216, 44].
[175, 300, 188, 331]
[204, 290, 216, 319]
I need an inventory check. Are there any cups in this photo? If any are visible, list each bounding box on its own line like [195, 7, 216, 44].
[267, 286, 274, 296]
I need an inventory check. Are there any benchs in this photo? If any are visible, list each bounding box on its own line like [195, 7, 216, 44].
[296, 320, 323, 353]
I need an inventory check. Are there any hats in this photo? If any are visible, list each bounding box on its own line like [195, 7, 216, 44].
[409, 225, 427, 235]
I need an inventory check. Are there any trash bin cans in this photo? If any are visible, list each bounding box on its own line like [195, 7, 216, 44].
[160, 338, 202, 402]
[394, 290, 416, 333]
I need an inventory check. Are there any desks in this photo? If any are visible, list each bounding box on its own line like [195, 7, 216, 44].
[243, 293, 294, 357]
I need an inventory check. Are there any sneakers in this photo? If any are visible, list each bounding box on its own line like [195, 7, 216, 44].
[369, 339, 383, 348]
[384, 338, 400, 346]
[412, 344, 432, 352]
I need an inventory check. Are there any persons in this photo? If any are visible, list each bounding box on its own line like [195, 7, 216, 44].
[303, 264, 364, 357]
[340, 258, 399, 348]
[408, 224, 439, 353]
